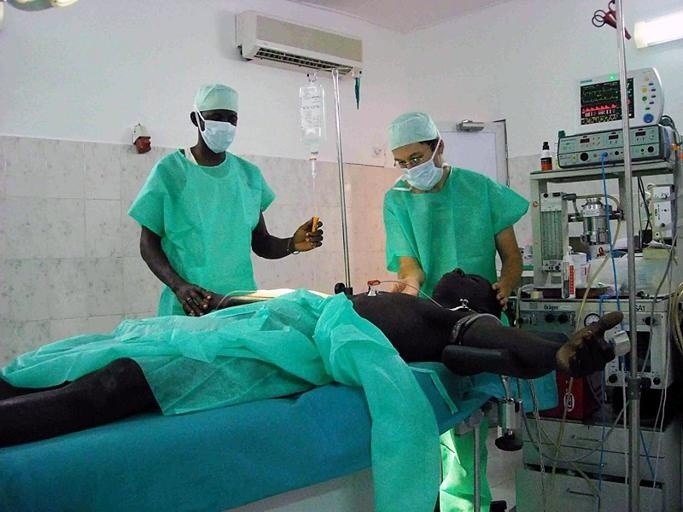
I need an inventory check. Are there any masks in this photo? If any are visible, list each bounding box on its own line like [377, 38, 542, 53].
[201, 120, 237, 155]
[400, 158, 444, 191]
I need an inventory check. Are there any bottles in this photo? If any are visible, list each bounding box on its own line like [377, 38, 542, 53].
[560, 254, 575, 300]
[540, 141, 552, 169]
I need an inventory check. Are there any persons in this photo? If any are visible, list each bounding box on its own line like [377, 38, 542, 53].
[127, 83, 323, 317]
[381, 111, 532, 511]
[0, 268, 625, 446]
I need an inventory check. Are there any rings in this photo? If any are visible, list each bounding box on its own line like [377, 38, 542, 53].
[185, 294, 194, 302]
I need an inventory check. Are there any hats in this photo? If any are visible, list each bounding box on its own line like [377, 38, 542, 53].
[389, 112, 439, 151]
[193, 83, 240, 113]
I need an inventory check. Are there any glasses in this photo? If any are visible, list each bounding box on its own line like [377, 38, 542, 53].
[393, 144, 431, 169]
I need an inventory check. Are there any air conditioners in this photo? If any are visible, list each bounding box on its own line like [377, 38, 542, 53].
[235, 10, 362, 83]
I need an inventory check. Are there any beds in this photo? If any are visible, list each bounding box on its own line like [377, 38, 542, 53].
[2, 356, 555, 510]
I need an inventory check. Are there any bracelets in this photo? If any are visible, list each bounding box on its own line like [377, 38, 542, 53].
[286, 238, 300, 256]
